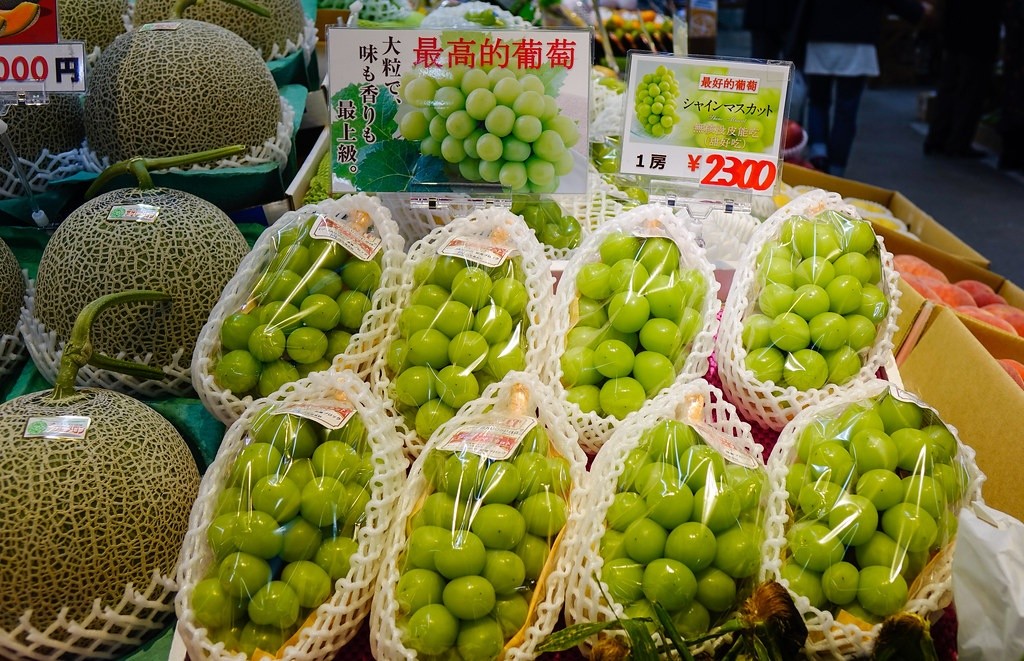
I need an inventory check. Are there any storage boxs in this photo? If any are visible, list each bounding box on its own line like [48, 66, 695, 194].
[0, 0, 1024, 661]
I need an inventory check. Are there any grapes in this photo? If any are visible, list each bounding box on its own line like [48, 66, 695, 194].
[633, 65, 780, 151]
[188, 139, 963, 660]
[394, 43, 585, 195]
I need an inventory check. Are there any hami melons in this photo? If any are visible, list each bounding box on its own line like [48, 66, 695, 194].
[0, 0, 308, 661]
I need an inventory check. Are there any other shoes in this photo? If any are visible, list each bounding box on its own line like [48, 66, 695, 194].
[922, 141, 939, 162]
[809, 143, 828, 169]
[826, 164, 844, 177]
[953, 147, 990, 160]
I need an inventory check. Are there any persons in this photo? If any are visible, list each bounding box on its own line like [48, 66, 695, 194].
[921, 0, 994, 161]
[732, 0, 1024, 178]
[794, 0, 882, 178]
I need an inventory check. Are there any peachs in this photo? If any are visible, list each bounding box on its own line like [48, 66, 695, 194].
[893, 253, 1024, 396]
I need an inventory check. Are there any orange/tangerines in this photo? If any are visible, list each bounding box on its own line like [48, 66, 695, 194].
[597, 10, 673, 35]
